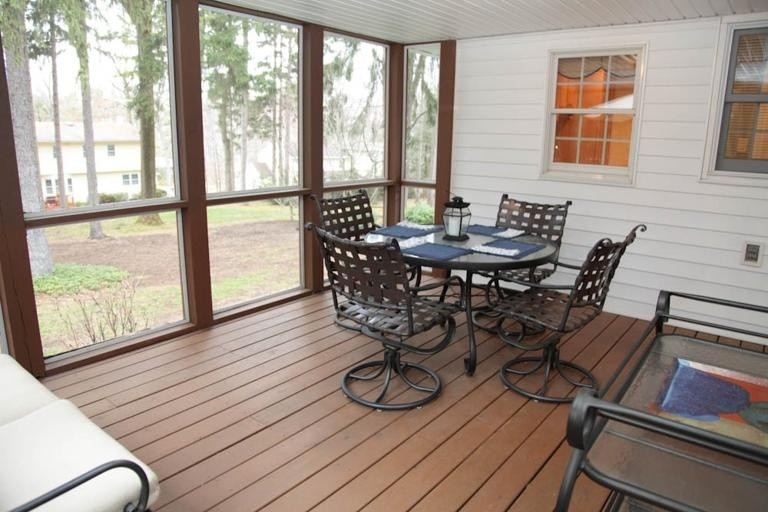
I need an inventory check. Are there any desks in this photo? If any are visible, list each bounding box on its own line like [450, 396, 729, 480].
[364, 224, 560, 376]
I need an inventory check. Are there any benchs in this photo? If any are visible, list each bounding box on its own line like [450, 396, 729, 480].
[0, 354, 162, 512]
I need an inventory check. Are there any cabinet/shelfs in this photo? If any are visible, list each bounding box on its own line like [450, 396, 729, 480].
[554, 290, 768, 511]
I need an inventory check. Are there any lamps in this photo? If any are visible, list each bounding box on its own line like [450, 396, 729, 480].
[441, 196, 471, 242]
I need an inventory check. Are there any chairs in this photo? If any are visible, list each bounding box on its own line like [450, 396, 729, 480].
[304, 221, 465, 412]
[478, 194, 572, 331]
[310, 187, 416, 283]
[492, 225, 647, 403]
